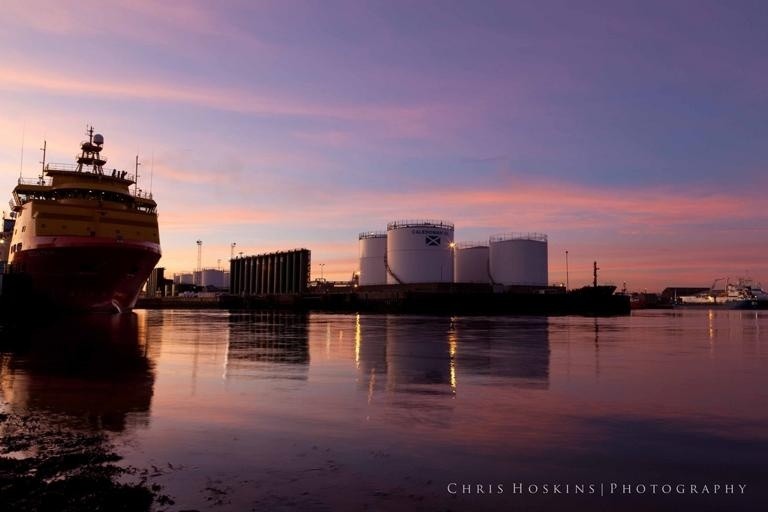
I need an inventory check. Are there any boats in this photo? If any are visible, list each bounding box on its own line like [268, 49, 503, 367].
[676, 276, 767, 305]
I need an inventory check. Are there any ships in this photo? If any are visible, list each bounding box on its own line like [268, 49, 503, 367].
[1, 123, 163, 325]
